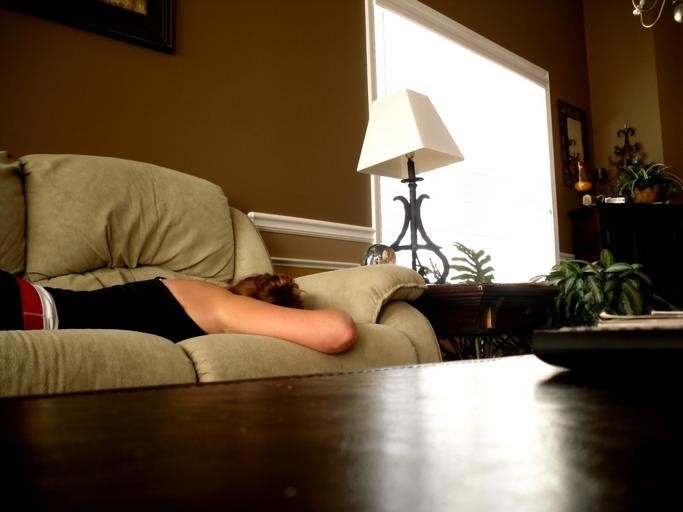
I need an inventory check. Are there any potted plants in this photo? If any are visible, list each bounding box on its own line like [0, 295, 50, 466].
[612, 159, 683, 205]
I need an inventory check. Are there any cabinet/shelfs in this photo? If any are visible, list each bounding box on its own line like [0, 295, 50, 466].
[569, 203, 682, 311]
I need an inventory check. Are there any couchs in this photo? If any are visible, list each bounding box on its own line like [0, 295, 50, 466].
[1, 149, 444, 400]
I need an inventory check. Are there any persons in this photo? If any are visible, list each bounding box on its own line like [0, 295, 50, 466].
[2, 271, 358, 355]
[373, 248, 395, 265]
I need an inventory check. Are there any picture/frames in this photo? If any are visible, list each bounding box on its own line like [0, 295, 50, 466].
[557, 99, 595, 189]
[0, 0, 178, 55]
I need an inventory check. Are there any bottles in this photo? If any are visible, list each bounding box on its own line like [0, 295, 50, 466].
[574, 160, 592, 191]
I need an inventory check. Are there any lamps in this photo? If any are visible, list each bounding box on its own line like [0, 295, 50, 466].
[629, 1, 683, 32]
[354, 88, 464, 286]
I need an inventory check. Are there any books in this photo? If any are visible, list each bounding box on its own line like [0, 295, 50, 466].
[596, 309, 682, 331]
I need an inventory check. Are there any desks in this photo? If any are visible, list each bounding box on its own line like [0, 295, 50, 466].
[403, 281, 561, 361]
[0, 353, 681, 511]
